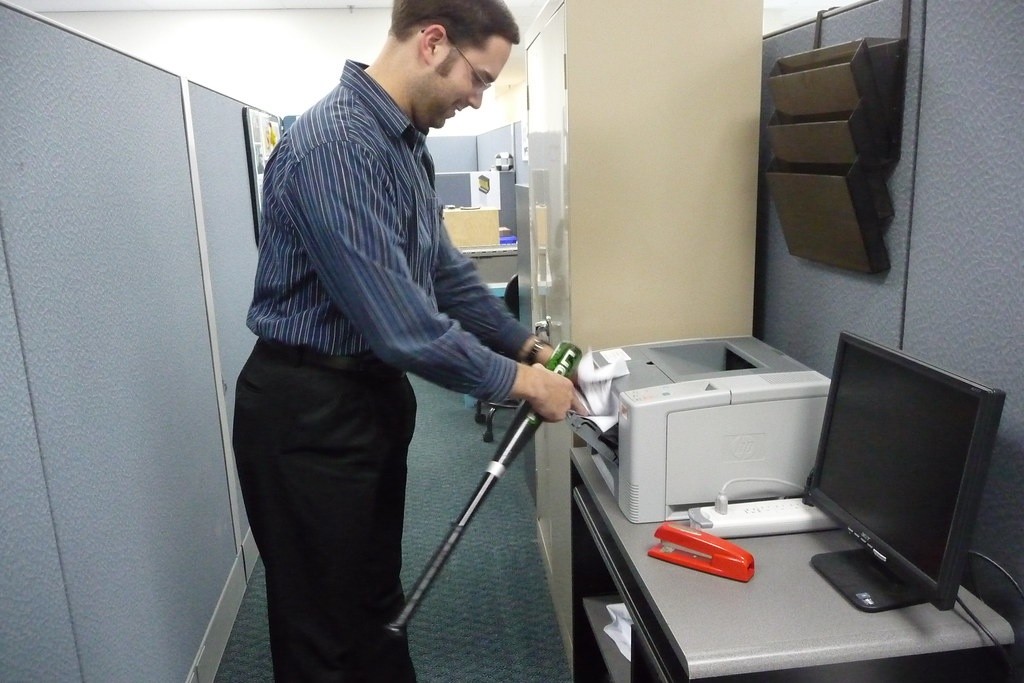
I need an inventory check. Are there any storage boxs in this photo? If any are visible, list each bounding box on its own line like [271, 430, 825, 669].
[441, 208, 500, 247]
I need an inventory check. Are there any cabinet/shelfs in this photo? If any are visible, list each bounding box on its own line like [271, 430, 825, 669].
[568, 448, 1018, 683]
[522, 0, 766, 683]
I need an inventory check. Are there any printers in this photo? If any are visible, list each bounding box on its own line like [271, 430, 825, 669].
[574, 335, 831, 523]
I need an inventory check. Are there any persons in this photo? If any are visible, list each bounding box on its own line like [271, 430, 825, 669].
[232, 0, 577, 683]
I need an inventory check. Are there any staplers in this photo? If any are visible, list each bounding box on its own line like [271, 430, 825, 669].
[646, 520, 757, 583]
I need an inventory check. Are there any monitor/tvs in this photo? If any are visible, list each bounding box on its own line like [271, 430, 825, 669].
[806, 329, 1006, 614]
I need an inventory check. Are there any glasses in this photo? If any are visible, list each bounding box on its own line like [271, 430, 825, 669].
[421, 27, 491, 97]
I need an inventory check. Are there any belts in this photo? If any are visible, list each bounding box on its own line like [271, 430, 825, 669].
[255, 333, 408, 382]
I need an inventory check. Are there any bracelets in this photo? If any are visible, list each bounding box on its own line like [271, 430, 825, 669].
[527, 339, 546, 363]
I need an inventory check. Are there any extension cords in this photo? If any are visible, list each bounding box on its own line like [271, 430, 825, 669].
[688, 496, 842, 538]
